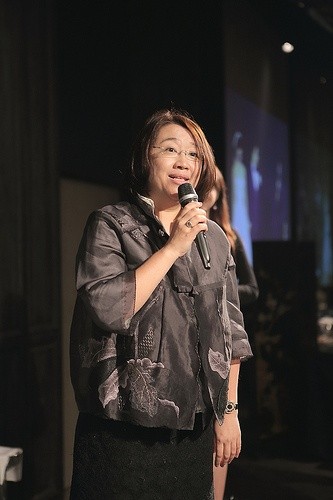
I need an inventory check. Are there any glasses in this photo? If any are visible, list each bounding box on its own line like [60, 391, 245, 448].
[147, 141, 200, 162]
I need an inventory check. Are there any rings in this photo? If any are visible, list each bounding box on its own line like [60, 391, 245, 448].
[187, 220, 193, 228]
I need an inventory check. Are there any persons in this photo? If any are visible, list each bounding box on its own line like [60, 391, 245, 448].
[199, 164, 259, 500]
[69, 111, 254, 500]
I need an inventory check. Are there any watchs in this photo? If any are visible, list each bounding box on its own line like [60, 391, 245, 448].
[222, 402, 239, 414]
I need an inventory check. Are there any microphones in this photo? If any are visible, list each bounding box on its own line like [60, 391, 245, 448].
[177, 183, 211, 270]
